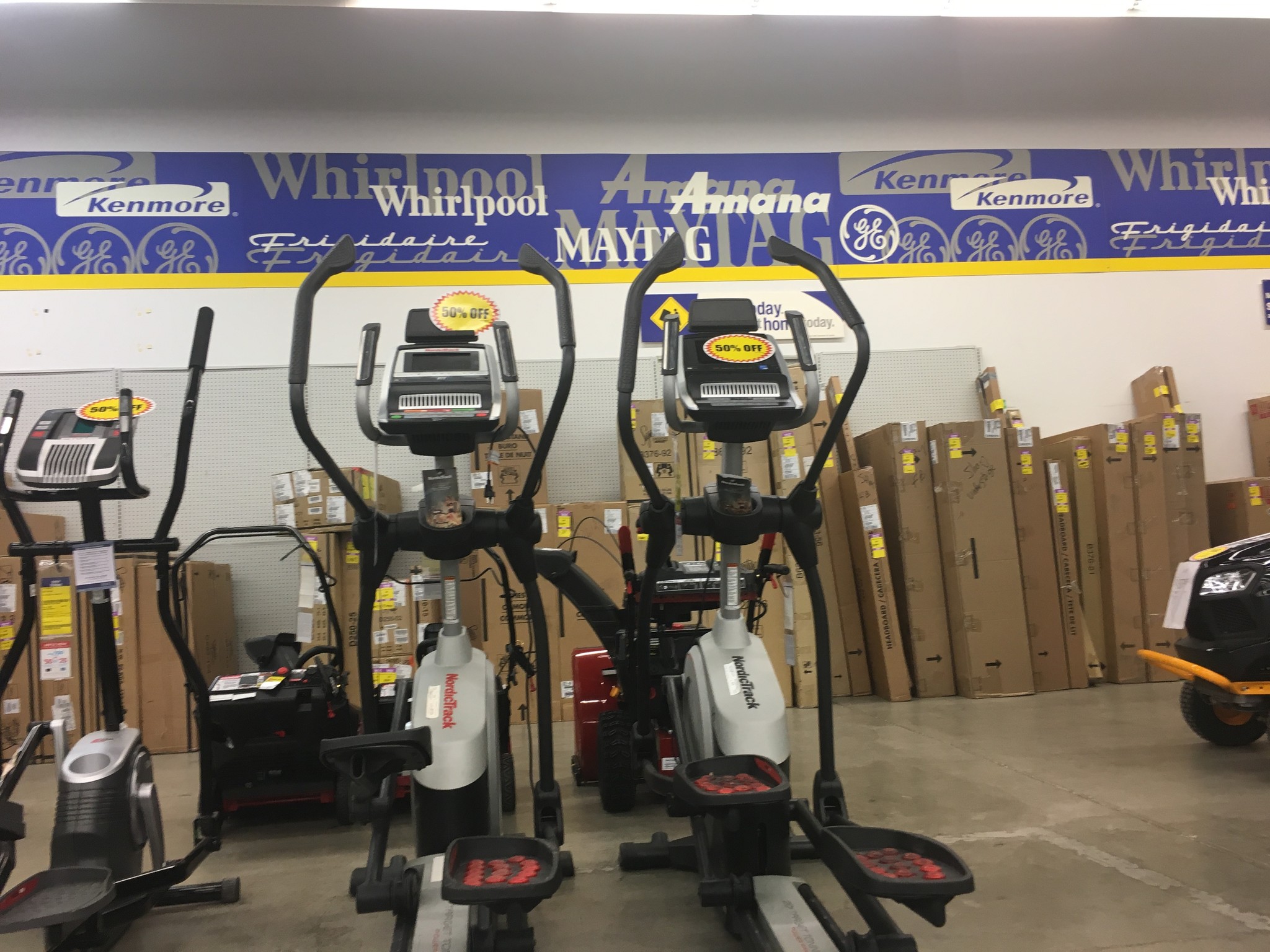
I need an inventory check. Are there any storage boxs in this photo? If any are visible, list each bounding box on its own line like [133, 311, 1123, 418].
[0, 365, 1270, 765]
